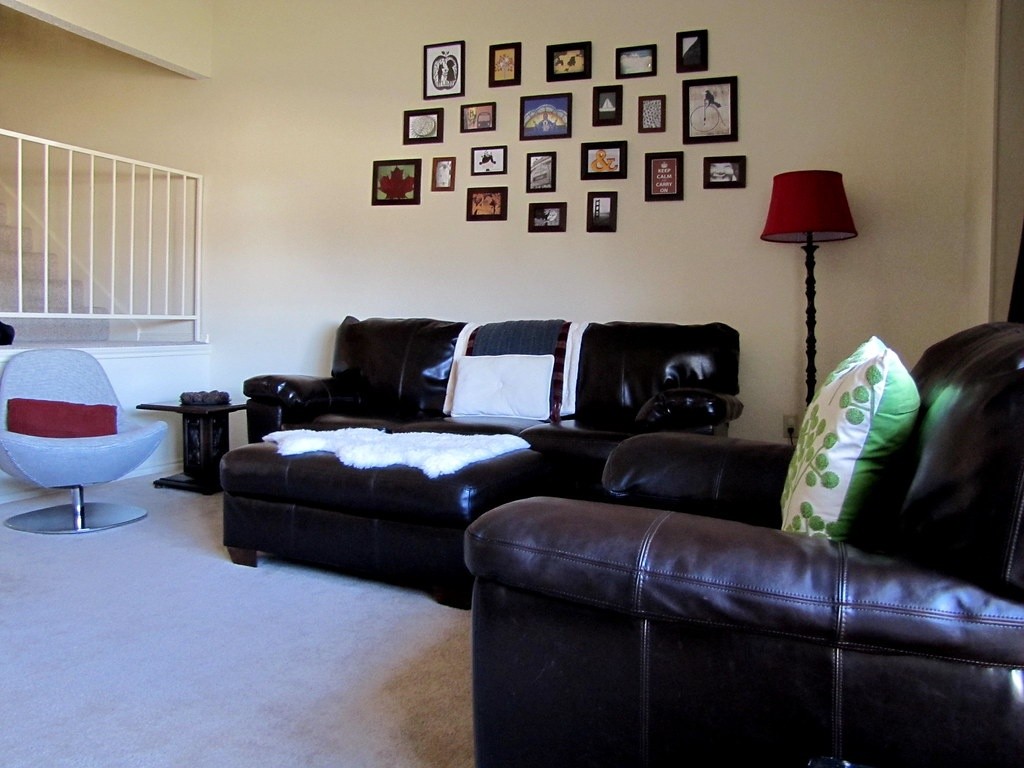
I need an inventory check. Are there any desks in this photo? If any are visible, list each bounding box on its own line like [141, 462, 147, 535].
[137, 400, 247, 496]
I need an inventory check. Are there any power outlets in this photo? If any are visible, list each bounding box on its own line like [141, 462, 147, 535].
[783, 415, 799, 439]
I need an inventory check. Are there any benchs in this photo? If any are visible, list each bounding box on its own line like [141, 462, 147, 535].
[220, 428, 544, 609]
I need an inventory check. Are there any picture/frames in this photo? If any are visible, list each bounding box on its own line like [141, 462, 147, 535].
[518, 92, 572, 141]
[703, 154, 748, 190]
[586, 191, 618, 233]
[676, 29, 708, 74]
[580, 141, 628, 181]
[371, 40, 522, 222]
[644, 150, 686, 202]
[637, 95, 666, 134]
[545, 40, 592, 83]
[681, 75, 738, 145]
[527, 152, 557, 194]
[527, 201, 567, 232]
[614, 44, 657, 79]
[592, 85, 624, 125]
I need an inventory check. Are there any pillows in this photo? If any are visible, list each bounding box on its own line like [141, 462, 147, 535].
[450, 353, 555, 421]
[7, 397, 118, 438]
[779, 336, 922, 542]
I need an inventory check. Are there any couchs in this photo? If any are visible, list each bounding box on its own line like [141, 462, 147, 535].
[459, 318, 1024, 768]
[218, 316, 744, 612]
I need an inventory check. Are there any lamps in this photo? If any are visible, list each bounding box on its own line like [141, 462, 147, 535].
[760, 170, 858, 405]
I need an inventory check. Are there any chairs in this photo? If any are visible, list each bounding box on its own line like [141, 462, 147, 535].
[0, 348, 168, 534]
